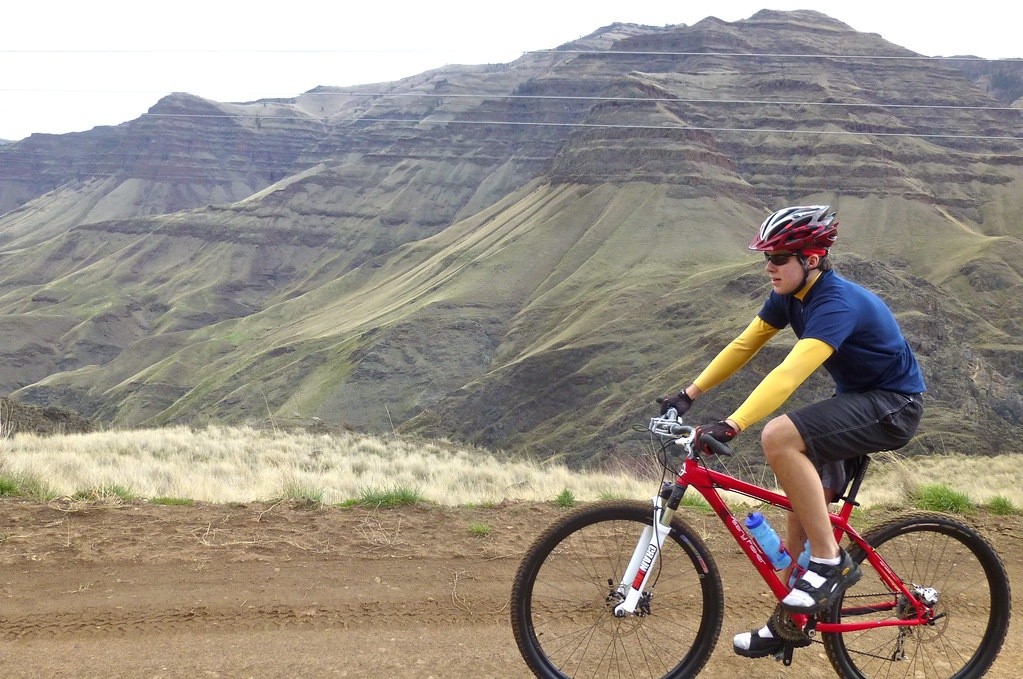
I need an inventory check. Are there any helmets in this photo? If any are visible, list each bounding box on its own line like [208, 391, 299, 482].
[748, 205, 839, 257]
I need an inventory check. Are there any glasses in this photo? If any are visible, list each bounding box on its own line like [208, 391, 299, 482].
[763, 251, 800, 266]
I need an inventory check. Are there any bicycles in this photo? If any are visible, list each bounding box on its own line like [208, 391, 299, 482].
[511, 397, 1011, 679]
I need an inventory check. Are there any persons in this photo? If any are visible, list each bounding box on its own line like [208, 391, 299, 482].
[660, 206, 927, 658]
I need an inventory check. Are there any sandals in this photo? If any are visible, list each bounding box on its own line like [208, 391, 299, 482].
[781, 546, 863, 613]
[733, 615, 812, 659]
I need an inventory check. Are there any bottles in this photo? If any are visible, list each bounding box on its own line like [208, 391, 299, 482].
[789, 535, 812, 590]
[744, 512, 792, 569]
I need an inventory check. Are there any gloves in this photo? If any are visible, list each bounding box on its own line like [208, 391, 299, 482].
[693, 420, 737, 456]
[660, 389, 695, 420]
